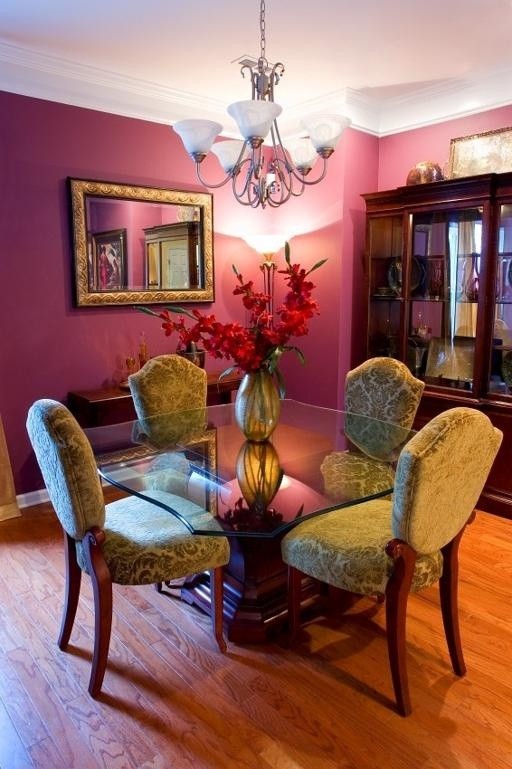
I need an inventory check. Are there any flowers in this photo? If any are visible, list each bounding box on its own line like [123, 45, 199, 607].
[130, 241, 329, 399]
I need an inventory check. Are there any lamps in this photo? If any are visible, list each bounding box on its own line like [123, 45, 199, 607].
[240, 235, 287, 330]
[170, 2, 351, 206]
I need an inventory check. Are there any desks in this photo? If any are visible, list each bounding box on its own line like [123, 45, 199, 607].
[70, 397, 429, 649]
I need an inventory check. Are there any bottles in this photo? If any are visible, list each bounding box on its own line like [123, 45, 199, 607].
[463, 254, 479, 300]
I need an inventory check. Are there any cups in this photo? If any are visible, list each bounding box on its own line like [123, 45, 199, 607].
[425, 259, 444, 297]
[378, 288, 388, 294]
[176, 350, 205, 370]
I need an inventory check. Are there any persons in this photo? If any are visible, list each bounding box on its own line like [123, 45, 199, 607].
[100, 244, 121, 290]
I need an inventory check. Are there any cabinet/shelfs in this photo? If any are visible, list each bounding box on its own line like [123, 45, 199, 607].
[138, 222, 202, 290]
[359, 170, 511, 519]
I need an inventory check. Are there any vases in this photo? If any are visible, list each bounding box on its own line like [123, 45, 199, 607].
[237, 437, 279, 507]
[232, 358, 283, 438]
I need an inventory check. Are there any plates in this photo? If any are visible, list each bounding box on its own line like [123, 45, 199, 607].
[386, 258, 423, 294]
[372, 294, 395, 298]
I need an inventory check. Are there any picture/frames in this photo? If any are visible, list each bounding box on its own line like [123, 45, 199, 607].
[449, 126, 512, 181]
[93, 227, 128, 291]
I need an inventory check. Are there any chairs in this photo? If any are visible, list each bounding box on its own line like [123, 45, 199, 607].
[136, 400, 208, 450]
[127, 354, 209, 418]
[24, 395, 232, 698]
[343, 355, 426, 429]
[278, 402, 504, 716]
[318, 442, 395, 501]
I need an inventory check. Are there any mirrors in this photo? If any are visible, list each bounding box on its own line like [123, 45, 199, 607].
[71, 177, 218, 306]
